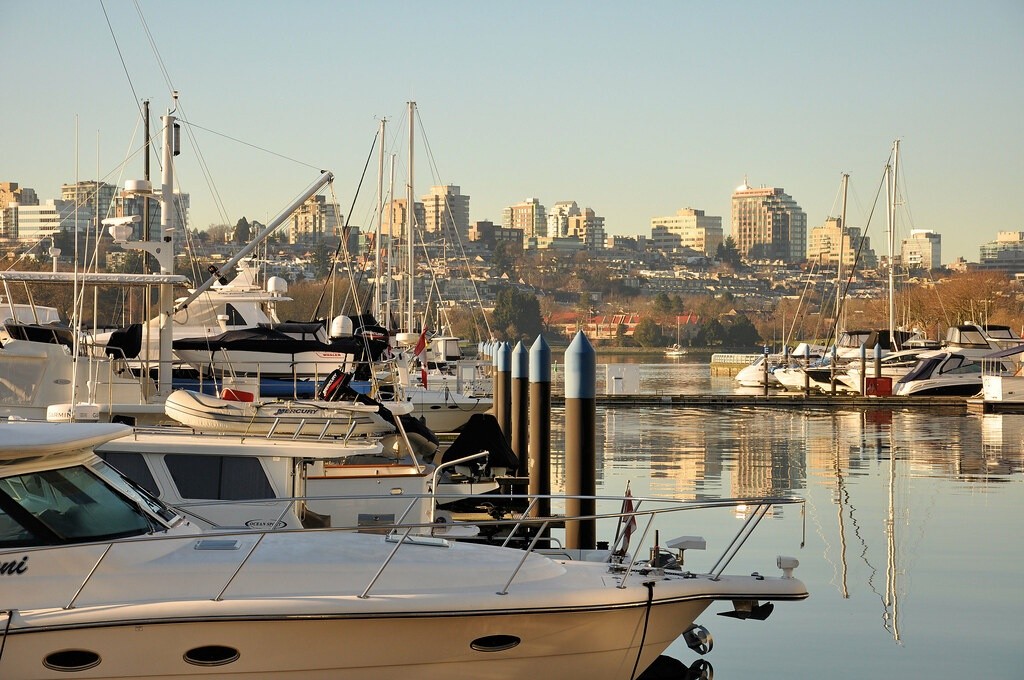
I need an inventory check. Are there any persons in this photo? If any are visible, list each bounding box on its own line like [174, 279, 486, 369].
[821, 340, 825, 346]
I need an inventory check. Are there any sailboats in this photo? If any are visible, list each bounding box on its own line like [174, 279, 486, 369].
[799, 164, 937, 393]
[833, 138, 994, 399]
[735, 172, 909, 387]
[2, 99, 574, 541]
[663, 317, 689, 356]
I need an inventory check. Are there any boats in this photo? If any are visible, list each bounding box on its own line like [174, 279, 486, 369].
[1, 420, 810, 679]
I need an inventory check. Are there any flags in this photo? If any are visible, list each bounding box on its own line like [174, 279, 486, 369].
[619, 490, 636, 557]
[416, 330, 427, 390]
